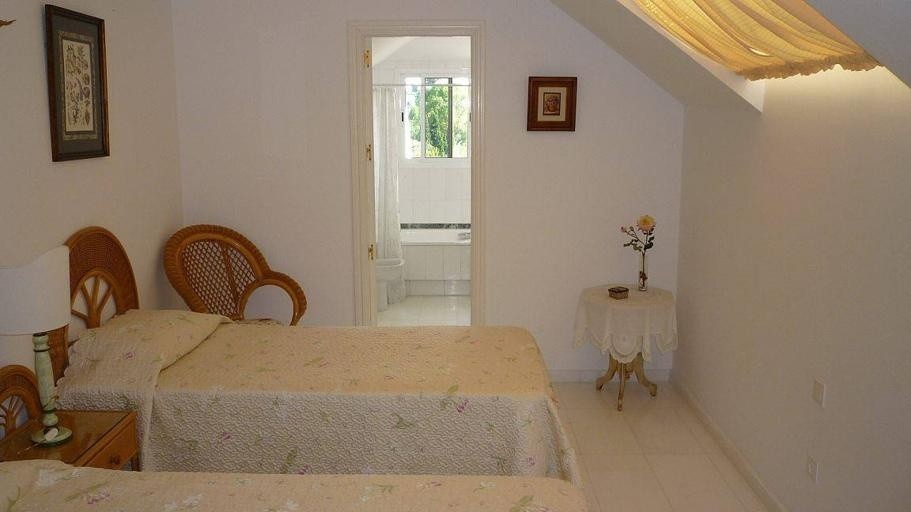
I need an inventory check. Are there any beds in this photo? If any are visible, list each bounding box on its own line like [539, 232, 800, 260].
[0, 458, 589, 512]
[46, 227, 581, 480]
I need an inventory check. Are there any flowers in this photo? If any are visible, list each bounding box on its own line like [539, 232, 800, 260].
[620, 215, 656, 286]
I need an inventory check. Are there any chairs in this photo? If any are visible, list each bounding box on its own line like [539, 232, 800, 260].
[164, 225, 308, 327]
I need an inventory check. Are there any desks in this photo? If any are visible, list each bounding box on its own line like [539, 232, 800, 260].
[575, 284, 675, 411]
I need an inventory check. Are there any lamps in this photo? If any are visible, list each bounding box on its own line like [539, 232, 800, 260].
[0, 246, 73, 448]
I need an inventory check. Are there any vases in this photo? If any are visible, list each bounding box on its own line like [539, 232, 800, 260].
[638, 251, 648, 290]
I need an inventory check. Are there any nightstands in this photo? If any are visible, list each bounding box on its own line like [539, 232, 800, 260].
[0, 365, 140, 473]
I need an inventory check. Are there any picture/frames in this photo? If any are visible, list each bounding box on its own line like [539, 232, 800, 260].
[46, 4, 110, 161]
[527, 76, 577, 131]
